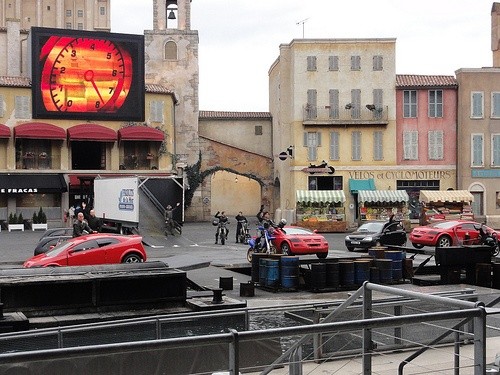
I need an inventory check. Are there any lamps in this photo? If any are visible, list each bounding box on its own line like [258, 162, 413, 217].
[344, 103, 376, 111]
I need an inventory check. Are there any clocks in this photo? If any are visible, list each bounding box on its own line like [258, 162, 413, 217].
[41, 36, 133, 113]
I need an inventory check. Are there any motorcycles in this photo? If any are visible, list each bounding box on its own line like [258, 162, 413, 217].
[247, 223, 282, 261]
[473, 224, 500, 258]
[166, 217, 173, 236]
[213, 219, 230, 245]
[240, 220, 251, 243]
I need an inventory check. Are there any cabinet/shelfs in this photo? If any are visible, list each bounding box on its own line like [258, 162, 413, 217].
[360, 201, 412, 233]
[296, 202, 347, 231]
[421, 201, 474, 225]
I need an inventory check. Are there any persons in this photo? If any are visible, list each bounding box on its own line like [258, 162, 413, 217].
[76, 205, 81, 212]
[235, 211, 248, 243]
[73, 213, 97, 239]
[256, 204, 287, 255]
[80, 202, 102, 233]
[69, 204, 75, 226]
[164, 203, 182, 236]
[212, 211, 230, 245]
[385, 212, 398, 230]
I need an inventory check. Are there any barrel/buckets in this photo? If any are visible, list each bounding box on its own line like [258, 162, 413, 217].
[311, 247, 412, 292]
[219, 276, 233, 290]
[240, 280, 254, 297]
[251, 252, 299, 292]
[475, 262, 500, 289]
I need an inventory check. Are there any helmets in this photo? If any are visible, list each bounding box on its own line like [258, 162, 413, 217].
[279, 219, 287, 228]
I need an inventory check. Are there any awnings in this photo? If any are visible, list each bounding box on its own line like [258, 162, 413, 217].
[67, 124, 117, 147]
[1, 172, 68, 194]
[348, 178, 377, 195]
[0, 124, 11, 139]
[418, 189, 475, 207]
[13, 122, 66, 144]
[117, 126, 165, 146]
[296, 189, 346, 204]
[358, 189, 410, 204]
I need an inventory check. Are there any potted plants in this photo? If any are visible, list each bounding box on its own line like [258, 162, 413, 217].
[32, 206, 47, 231]
[8, 212, 25, 232]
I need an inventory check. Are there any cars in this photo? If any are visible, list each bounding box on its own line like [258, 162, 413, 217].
[270, 226, 329, 259]
[345, 220, 408, 252]
[34, 227, 90, 255]
[23, 233, 146, 269]
[408, 219, 500, 248]
